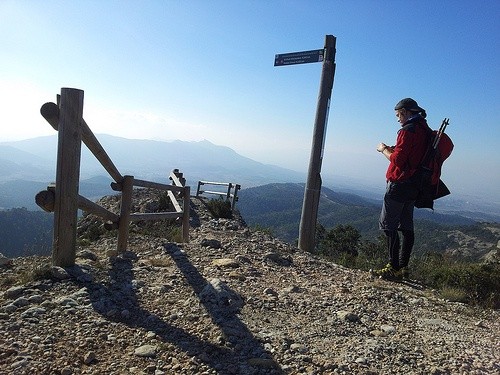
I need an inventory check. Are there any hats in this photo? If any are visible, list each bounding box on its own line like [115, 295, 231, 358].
[394, 97, 424, 111]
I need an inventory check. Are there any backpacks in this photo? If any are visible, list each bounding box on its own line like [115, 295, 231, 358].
[417, 119, 454, 211]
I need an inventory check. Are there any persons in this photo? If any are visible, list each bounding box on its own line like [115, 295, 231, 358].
[369, 97, 433, 282]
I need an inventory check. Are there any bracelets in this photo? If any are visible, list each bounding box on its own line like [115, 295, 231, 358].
[382, 147, 388, 154]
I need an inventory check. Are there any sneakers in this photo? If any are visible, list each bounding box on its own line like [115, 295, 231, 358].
[375, 262, 403, 280]
[399, 266, 410, 277]
